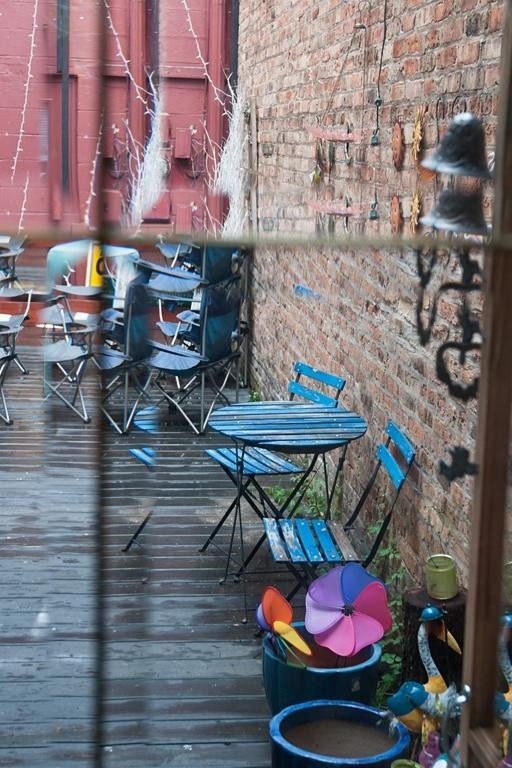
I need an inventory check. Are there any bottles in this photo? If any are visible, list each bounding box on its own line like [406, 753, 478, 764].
[424, 553, 457, 600]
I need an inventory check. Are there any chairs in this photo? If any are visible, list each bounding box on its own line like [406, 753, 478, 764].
[201, 363, 344, 582]
[255, 424, 414, 635]
[1, 238, 247, 434]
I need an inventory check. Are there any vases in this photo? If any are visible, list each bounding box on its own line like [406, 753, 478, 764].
[247, 619, 383, 711]
[272, 701, 410, 768]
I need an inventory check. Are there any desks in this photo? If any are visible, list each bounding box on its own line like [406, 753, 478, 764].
[210, 404, 366, 626]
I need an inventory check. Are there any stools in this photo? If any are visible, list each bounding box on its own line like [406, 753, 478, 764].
[403, 584, 468, 679]
